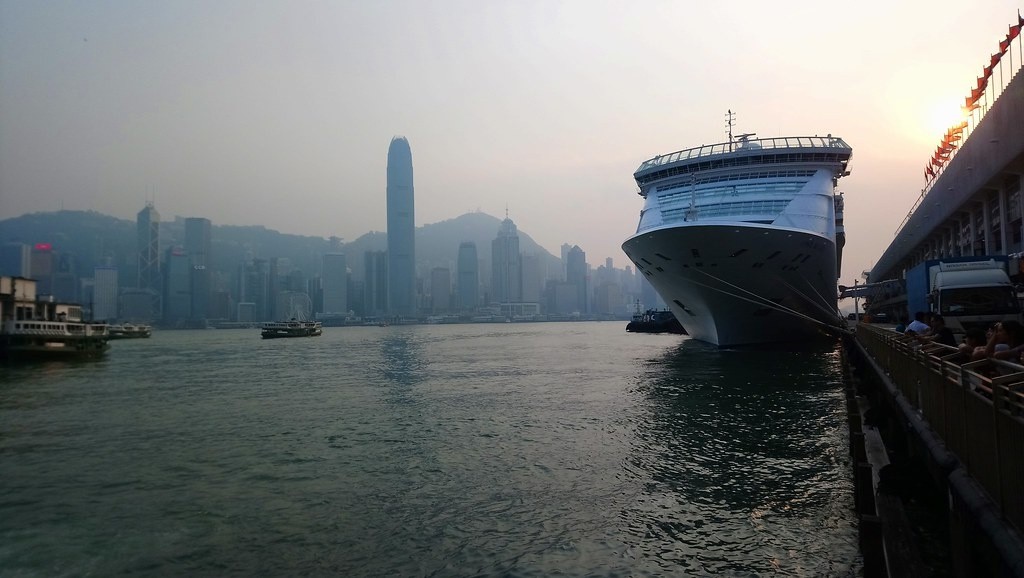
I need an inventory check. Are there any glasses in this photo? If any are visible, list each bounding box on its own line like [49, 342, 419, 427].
[986, 329, 993, 332]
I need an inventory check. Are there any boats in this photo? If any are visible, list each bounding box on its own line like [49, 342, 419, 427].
[625, 306, 683, 334]
[261, 318, 324, 338]
[0, 275, 113, 352]
[108, 320, 154, 339]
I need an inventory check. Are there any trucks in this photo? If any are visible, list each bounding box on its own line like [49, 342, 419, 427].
[903, 255, 1012, 341]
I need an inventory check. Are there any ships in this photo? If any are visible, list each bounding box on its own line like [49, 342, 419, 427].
[619, 104, 854, 348]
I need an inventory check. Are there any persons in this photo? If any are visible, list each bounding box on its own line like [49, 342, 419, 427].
[895, 309, 1024, 367]
[863, 312, 872, 323]
